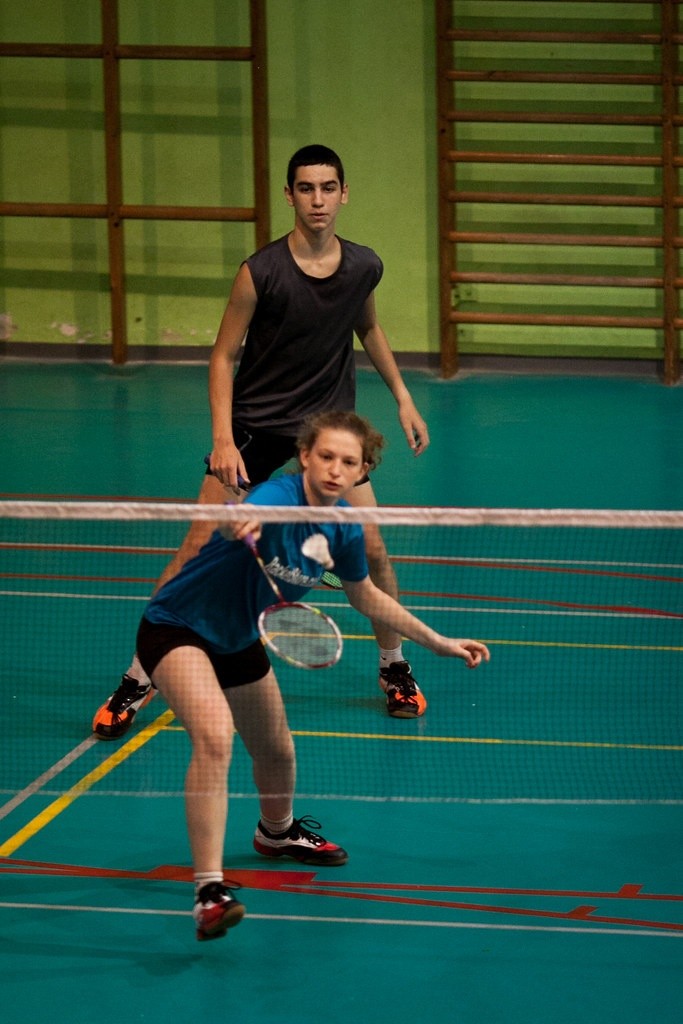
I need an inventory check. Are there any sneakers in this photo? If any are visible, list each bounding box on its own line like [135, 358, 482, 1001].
[377, 658, 427, 720]
[91, 672, 160, 741]
[252, 814, 350, 866]
[190, 879, 246, 942]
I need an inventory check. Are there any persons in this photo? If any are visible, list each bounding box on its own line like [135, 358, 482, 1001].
[90, 146, 433, 738]
[134, 409, 491, 941]
